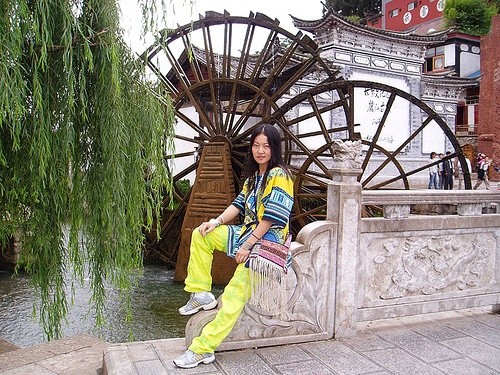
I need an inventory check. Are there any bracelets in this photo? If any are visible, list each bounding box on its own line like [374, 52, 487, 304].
[241, 240, 253, 252]
[252, 233, 259, 240]
[208, 215, 224, 227]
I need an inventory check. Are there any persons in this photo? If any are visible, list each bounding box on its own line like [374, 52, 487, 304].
[427, 151, 440, 189]
[473, 156, 492, 190]
[476, 154, 485, 169]
[438, 152, 445, 189]
[457, 153, 472, 190]
[443, 151, 454, 190]
[172, 123, 295, 368]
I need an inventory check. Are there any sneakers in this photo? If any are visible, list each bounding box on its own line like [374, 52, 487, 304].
[173, 349, 216, 369]
[178, 292, 218, 315]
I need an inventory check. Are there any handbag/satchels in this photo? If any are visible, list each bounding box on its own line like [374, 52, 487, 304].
[248, 237, 291, 322]
